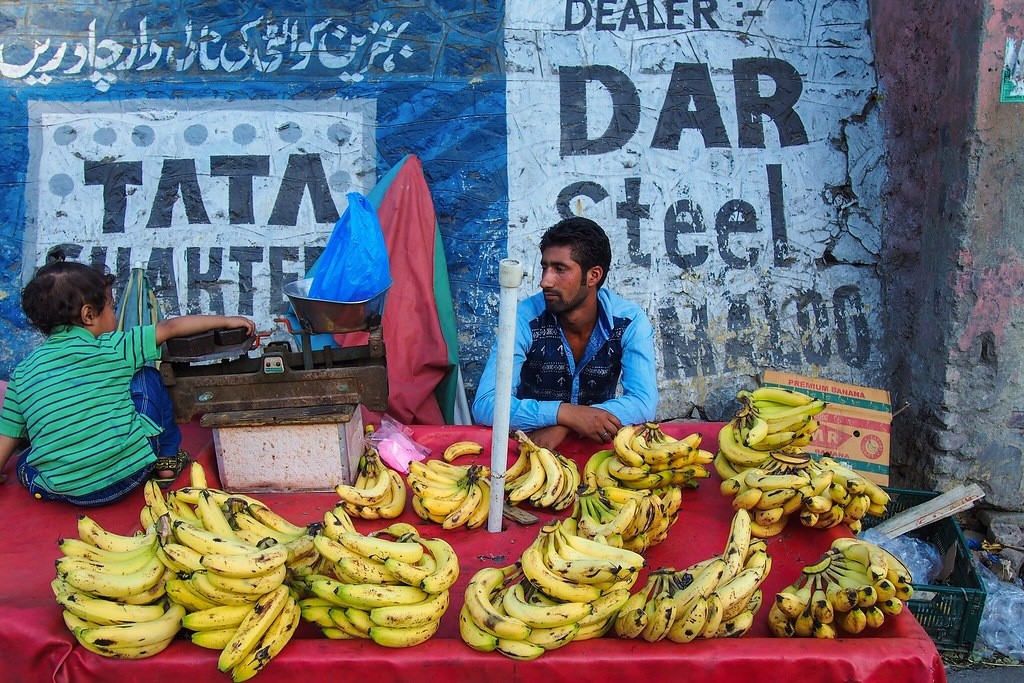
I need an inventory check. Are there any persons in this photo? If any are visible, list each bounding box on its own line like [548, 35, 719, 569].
[473, 216, 659, 453]
[0, 261, 255, 508]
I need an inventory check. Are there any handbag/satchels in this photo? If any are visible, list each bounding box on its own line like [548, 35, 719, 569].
[307, 188, 390, 303]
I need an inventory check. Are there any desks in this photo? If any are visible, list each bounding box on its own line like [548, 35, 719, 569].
[0, 416, 947, 683]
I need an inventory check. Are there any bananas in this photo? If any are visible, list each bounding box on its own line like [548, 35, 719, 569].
[459, 387, 914, 660]
[50, 439, 491, 683]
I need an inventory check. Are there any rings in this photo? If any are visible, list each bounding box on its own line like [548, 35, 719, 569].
[602, 432, 607, 437]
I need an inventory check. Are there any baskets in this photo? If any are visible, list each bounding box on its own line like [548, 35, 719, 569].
[843, 486, 987, 662]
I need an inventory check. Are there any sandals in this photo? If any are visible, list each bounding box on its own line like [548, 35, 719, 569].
[150, 448, 192, 488]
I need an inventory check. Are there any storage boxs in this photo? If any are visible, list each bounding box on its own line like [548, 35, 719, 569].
[736, 367, 895, 486]
[203, 401, 372, 494]
[861, 488, 988, 655]
